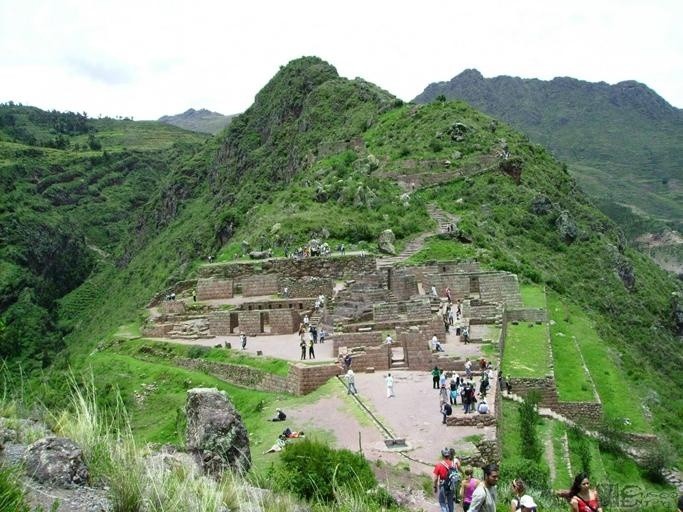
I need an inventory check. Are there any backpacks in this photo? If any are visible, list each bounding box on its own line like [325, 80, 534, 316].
[439, 460, 461, 491]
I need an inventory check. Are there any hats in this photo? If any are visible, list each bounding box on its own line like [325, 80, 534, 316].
[519, 494, 537, 508]
[441, 448, 450, 456]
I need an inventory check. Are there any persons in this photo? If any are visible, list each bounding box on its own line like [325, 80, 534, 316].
[239, 331, 244, 346]
[263, 427, 305, 453]
[384, 334, 394, 349]
[505, 373, 513, 394]
[283, 238, 346, 257]
[431, 284, 472, 352]
[191, 288, 197, 302]
[297, 292, 326, 360]
[498, 370, 503, 391]
[384, 373, 397, 399]
[430, 356, 496, 425]
[431, 446, 538, 512]
[344, 369, 358, 394]
[267, 407, 286, 421]
[242, 334, 246, 350]
[343, 353, 353, 372]
[567, 471, 603, 512]
[159, 289, 175, 301]
